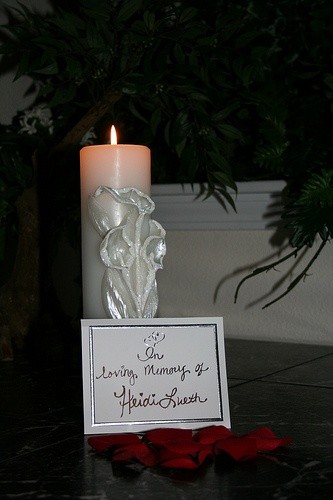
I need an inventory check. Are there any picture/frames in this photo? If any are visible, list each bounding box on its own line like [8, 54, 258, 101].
[79, 317, 232, 435]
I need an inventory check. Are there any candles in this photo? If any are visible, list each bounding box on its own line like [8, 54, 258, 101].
[78, 123, 167, 319]
